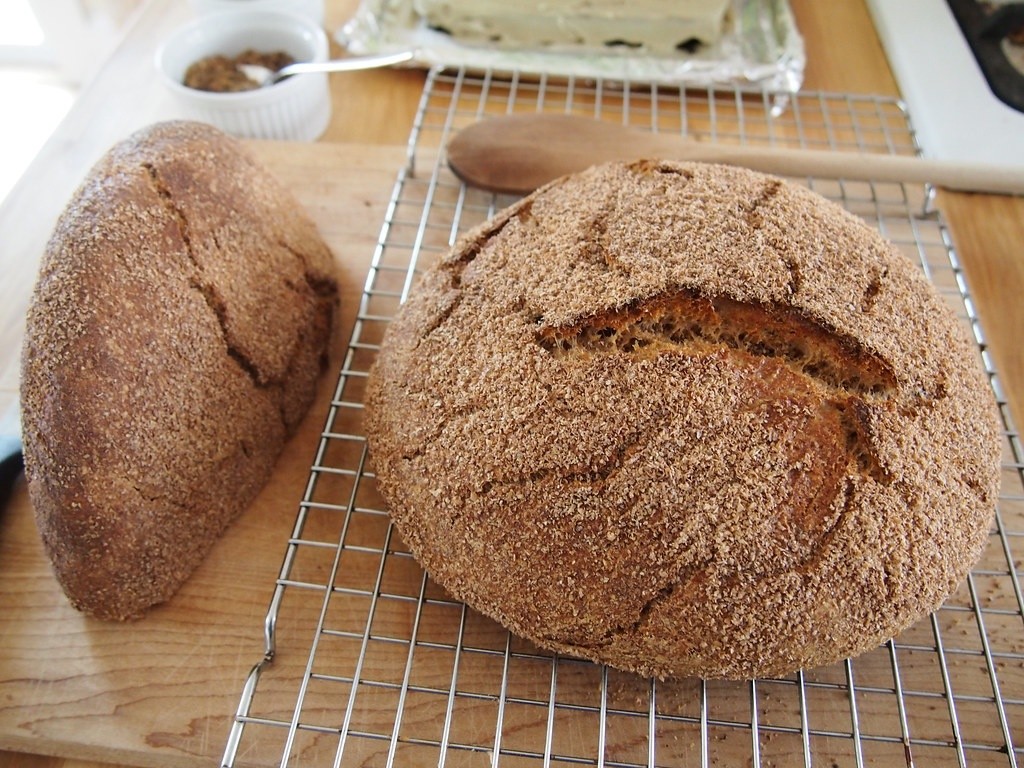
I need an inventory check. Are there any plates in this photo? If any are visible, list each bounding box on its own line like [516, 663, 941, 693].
[334, 0, 806, 94]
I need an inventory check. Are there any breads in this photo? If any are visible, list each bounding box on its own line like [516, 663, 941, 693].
[365, 159, 1000, 683]
[19, 123, 341, 624]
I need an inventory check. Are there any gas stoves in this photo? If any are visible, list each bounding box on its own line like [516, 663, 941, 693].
[866, 0, 1024, 195]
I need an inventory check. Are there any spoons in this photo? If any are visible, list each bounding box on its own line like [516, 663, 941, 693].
[446, 113, 1024, 193]
[237, 52, 413, 88]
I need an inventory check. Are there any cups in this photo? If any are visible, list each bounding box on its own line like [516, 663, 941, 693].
[157, 9, 332, 143]
